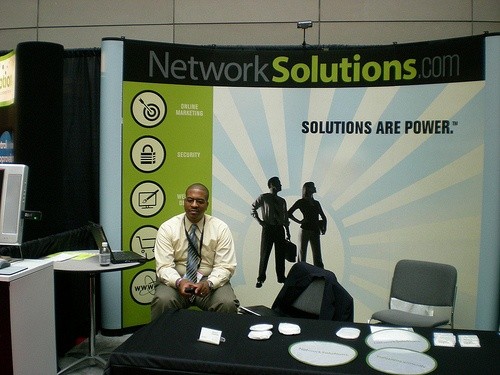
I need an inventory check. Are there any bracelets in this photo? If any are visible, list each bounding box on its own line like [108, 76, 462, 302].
[176, 278, 183, 290]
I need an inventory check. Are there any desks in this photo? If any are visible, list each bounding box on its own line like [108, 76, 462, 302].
[101, 310, 500, 375]
[47, 250, 145, 375]
[0, 256, 57, 375]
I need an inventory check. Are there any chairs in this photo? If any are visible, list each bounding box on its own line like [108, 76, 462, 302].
[367, 259, 457, 329]
[238, 262, 354, 321]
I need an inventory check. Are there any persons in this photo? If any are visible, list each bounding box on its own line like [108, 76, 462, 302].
[251, 177, 291, 288]
[287, 181, 327, 270]
[150, 184, 240, 325]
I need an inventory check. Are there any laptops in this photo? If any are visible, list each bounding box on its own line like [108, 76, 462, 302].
[88, 221, 147, 264]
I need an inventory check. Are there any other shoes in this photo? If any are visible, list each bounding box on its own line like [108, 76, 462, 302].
[256, 277, 264, 288]
[278, 276, 286, 283]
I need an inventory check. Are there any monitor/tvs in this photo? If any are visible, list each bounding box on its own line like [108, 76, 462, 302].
[0, 163, 29, 246]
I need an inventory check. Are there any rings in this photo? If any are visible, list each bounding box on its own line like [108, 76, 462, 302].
[200, 293, 203, 295]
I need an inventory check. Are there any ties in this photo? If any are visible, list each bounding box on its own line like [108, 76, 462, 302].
[185, 224, 199, 302]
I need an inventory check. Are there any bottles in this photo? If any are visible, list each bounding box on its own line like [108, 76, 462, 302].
[100, 242, 110, 266]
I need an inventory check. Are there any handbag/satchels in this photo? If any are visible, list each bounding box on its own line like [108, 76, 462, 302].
[284, 237, 297, 263]
[318, 219, 325, 235]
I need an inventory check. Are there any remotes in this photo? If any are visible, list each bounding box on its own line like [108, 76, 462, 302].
[185, 286, 197, 294]
